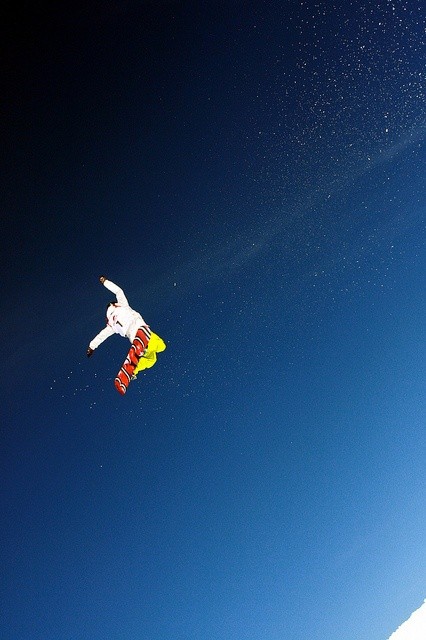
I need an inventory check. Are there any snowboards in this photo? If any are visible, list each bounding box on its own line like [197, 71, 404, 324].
[113, 326, 152, 396]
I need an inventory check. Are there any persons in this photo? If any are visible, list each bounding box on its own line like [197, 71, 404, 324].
[85, 276, 165, 382]
